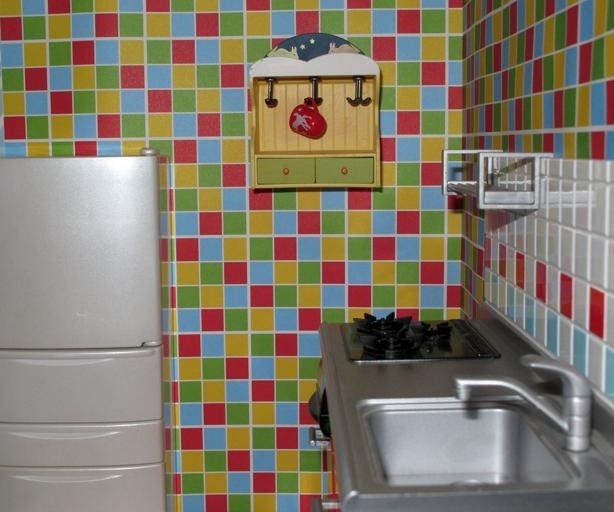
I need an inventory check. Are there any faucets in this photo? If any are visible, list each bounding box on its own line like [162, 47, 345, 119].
[451, 353, 593, 452]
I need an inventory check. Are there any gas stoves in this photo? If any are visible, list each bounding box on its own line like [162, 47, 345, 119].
[339, 313, 500, 363]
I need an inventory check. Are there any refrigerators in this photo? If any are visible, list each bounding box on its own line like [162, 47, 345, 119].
[1, 151, 168, 506]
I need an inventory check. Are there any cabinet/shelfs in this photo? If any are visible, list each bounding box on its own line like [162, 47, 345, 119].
[251, 74, 379, 189]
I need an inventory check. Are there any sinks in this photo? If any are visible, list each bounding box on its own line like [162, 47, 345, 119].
[328, 380, 614, 512]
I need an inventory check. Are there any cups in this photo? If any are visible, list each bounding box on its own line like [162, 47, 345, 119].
[288, 97, 327, 140]
